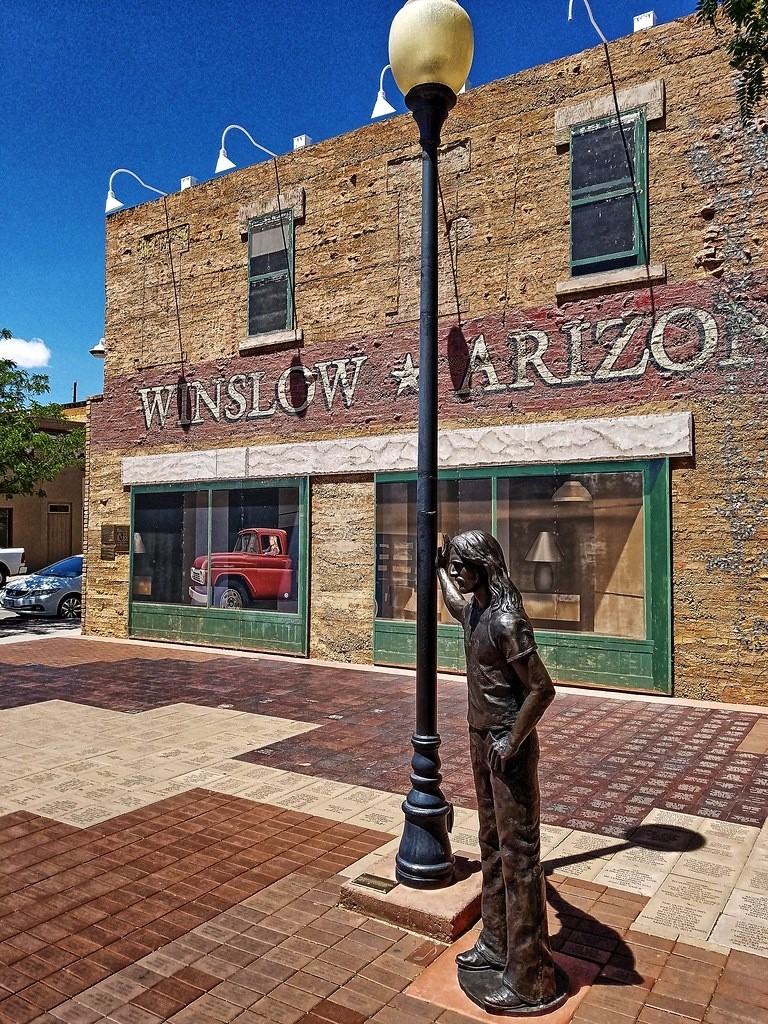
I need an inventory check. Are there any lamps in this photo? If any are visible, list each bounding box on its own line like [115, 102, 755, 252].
[105, 169, 168, 213]
[370, 64, 397, 118]
[214, 124, 278, 174]
[550, 480, 594, 503]
[89, 338, 105, 358]
[524, 531, 565, 592]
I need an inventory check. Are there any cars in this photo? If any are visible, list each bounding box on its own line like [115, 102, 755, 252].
[0, 554, 84, 619]
[0, 548, 28, 589]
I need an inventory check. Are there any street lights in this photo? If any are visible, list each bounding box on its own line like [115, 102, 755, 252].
[388, 0, 474, 890]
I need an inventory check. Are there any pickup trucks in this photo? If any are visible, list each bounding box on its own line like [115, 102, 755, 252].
[189, 528, 293, 609]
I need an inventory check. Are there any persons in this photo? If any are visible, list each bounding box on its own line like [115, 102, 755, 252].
[434, 529, 555, 1010]
[262, 536, 279, 557]
